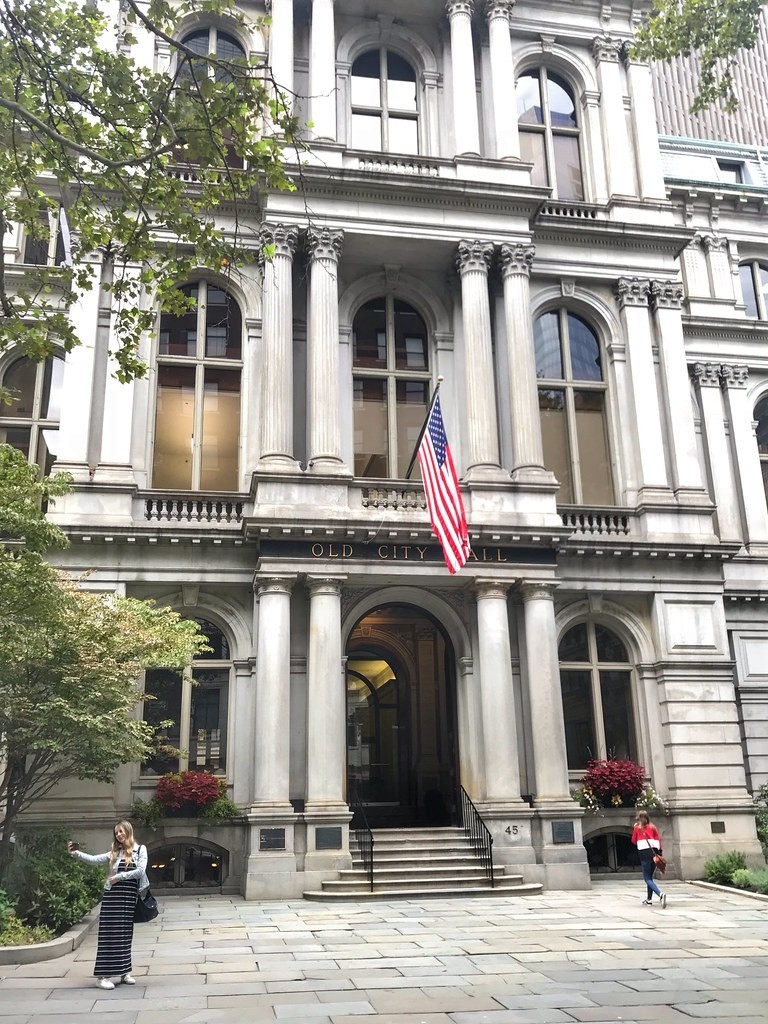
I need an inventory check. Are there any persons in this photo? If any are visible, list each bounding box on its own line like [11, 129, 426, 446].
[631, 810, 667, 909]
[68, 819, 148, 989]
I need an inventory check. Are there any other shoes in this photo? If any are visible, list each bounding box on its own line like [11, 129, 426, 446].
[122, 974, 136, 985]
[641, 900, 652, 906]
[96, 978, 115, 989]
[660, 893, 666, 909]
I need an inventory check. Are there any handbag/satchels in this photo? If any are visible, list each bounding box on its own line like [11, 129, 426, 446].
[653, 854, 667, 874]
[134, 891, 158, 923]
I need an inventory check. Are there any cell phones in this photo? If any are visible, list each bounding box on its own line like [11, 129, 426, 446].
[70, 842, 80, 851]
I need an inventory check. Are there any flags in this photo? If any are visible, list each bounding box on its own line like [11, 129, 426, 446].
[417, 395, 471, 575]
